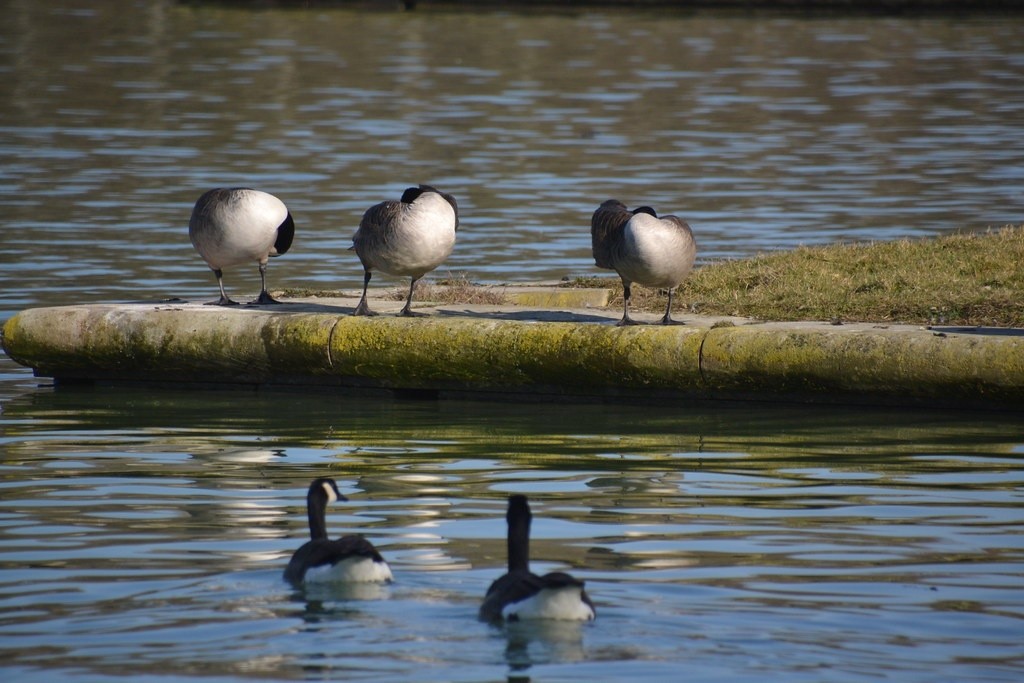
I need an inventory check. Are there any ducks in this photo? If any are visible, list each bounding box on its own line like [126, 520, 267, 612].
[348, 183, 459, 318]
[475, 494, 596, 623]
[284, 478, 393, 584]
[592, 199, 697, 325]
[189, 187, 295, 305]
